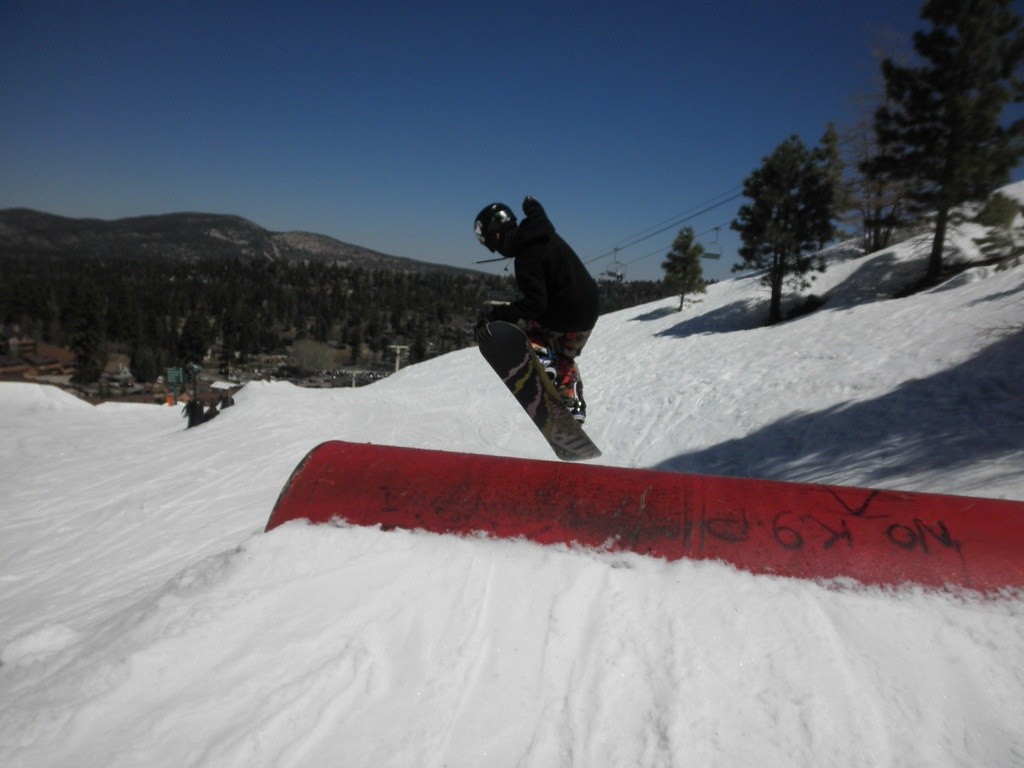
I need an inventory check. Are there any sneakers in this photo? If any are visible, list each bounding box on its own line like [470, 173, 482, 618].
[568, 406, 587, 423]
[544, 363, 558, 383]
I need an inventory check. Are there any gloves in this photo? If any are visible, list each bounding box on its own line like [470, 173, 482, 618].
[473, 317, 493, 343]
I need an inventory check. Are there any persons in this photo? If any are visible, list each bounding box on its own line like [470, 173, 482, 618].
[474, 196, 600, 423]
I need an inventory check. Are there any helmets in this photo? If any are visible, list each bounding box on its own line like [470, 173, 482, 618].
[474, 202, 518, 253]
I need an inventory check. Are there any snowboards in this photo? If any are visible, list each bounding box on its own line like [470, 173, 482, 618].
[475, 319, 604, 462]
